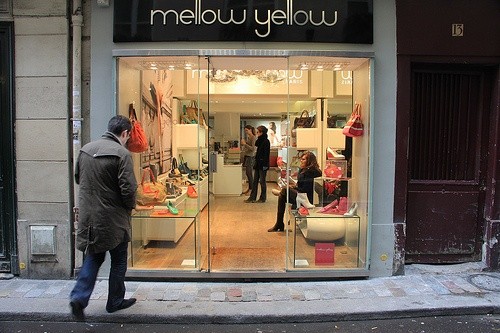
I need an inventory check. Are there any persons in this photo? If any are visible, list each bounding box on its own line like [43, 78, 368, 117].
[68, 116, 138, 321]
[241, 125, 256, 196]
[267, 152, 322, 232]
[243, 126, 270, 203]
[267, 122, 279, 145]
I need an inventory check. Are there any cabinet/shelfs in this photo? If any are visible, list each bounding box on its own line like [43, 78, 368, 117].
[277, 99, 346, 241]
[141, 99, 208, 246]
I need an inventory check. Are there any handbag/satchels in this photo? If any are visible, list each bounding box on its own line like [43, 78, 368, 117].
[125, 103, 148, 153]
[343, 103, 364, 138]
[294, 110, 315, 129]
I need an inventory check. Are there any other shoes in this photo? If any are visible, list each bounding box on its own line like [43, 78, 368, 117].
[243, 196, 257, 203]
[107, 297, 137, 313]
[70, 299, 85, 322]
[242, 189, 252, 196]
[256, 198, 266, 203]
[135, 152, 209, 215]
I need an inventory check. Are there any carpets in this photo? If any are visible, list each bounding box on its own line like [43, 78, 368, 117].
[202, 246, 293, 271]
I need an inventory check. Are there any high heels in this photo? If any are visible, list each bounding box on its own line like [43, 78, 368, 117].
[272, 189, 280, 196]
[317, 196, 359, 217]
[268, 222, 284, 232]
[296, 192, 315, 209]
